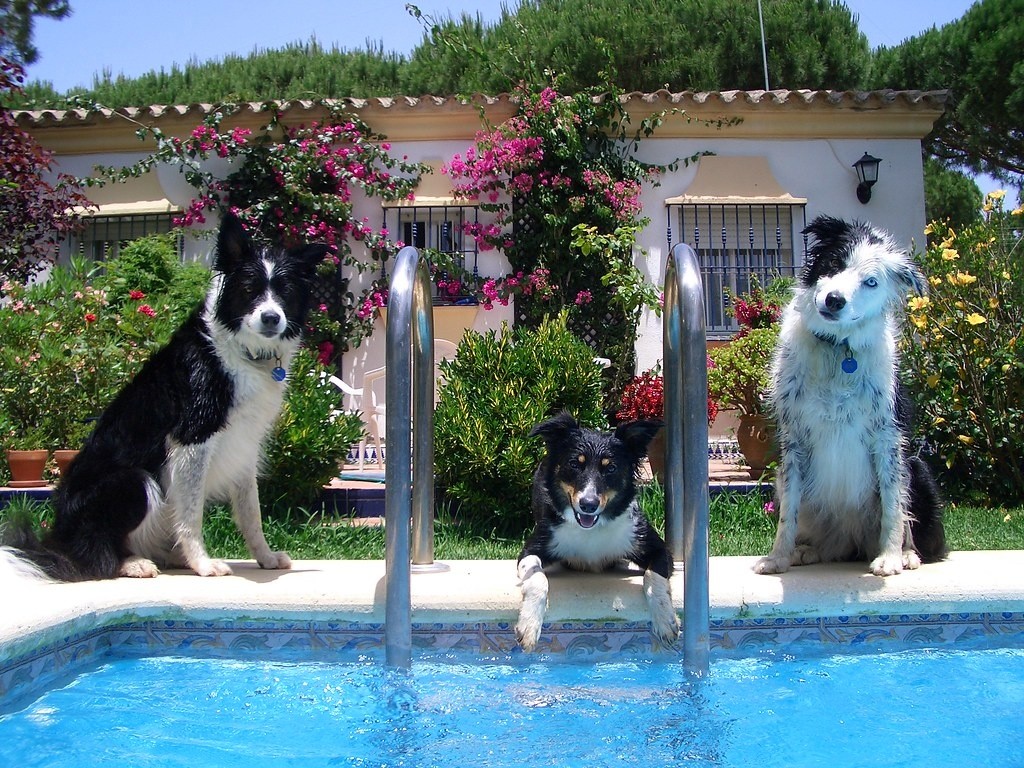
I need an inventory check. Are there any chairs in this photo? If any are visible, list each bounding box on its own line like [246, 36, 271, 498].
[307, 370, 384, 471]
[358, 338, 459, 473]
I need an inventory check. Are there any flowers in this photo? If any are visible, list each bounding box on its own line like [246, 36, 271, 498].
[0, 416, 61, 488]
[616, 367, 720, 428]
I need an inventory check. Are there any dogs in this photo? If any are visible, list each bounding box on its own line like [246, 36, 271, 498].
[1, 203, 327, 579]
[754, 211, 951, 575]
[513, 407, 678, 653]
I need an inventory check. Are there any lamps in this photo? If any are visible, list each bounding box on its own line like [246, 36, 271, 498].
[851, 152, 883, 204]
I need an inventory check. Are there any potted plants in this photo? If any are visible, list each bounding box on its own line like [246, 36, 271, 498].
[709, 324, 784, 480]
[54, 420, 96, 473]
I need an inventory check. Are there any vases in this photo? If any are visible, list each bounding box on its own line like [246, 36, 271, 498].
[379, 303, 479, 360]
[4, 450, 49, 481]
[646, 426, 666, 484]
